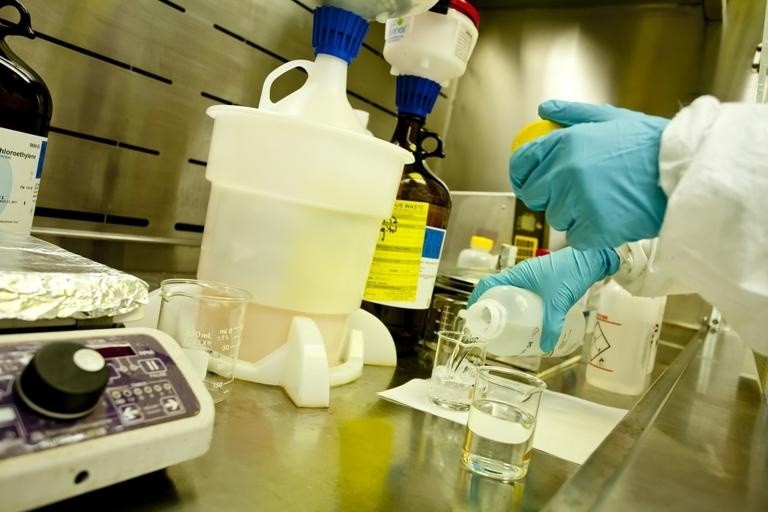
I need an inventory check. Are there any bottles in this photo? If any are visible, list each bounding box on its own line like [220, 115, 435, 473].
[457, 234, 497, 271]
[466, 285, 582, 356]
[581, 284, 665, 396]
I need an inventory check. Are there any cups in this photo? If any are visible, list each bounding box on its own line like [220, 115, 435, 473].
[465, 368, 542, 483]
[430, 328, 488, 408]
[425, 292, 472, 363]
[156, 277, 251, 401]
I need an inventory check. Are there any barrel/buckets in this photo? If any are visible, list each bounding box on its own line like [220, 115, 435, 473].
[195, 54, 416, 363]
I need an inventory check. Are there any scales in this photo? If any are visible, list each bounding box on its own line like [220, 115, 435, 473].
[0, 230, 215, 512]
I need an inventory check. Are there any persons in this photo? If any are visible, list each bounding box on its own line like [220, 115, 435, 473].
[467, 92, 767, 363]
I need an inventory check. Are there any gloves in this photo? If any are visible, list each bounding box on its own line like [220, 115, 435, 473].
[508, 98, 671, 250]
[466, 243, 620, 356]
